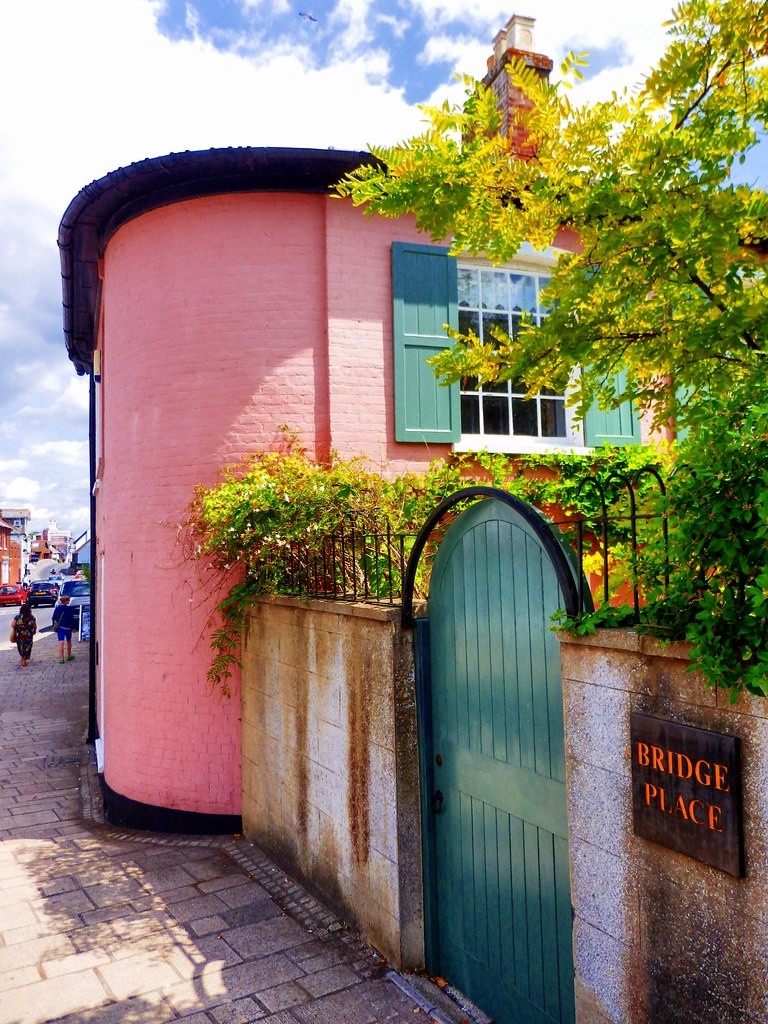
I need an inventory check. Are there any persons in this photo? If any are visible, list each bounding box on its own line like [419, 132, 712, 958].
[52, 596, 76, 664]
[11, 604, 37, 666]
[22, 573, 28, 590]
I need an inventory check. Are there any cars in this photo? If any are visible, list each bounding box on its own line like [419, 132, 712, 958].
[27, 580, 59, 608]
[44, 575, 65, 586]
[0, 582, 29, 606]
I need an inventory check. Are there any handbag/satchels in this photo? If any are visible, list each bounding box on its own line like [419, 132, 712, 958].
[53, 621, 59, 632]
[10, 615, 18, 643]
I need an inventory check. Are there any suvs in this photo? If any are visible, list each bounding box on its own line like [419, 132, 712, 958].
[53, 580, 91, 624]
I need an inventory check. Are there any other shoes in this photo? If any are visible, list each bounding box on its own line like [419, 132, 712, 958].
[66, 654, 75, 661]
[21, 658, 26, 666]
[59, 660, 64, 664]
